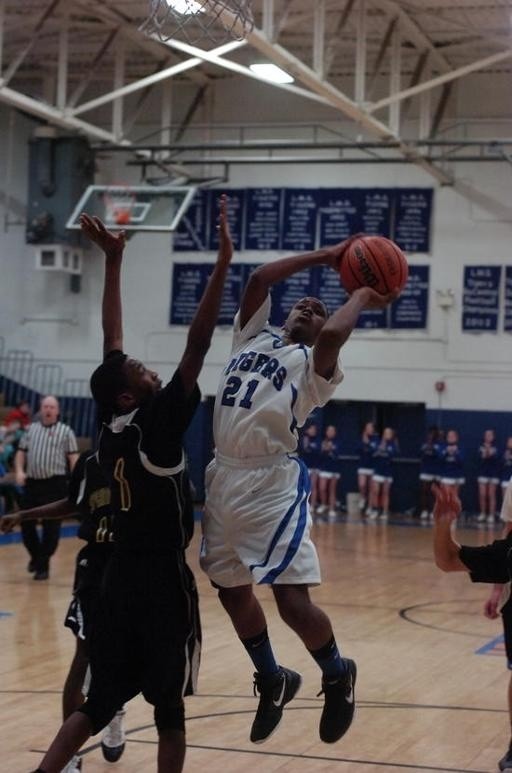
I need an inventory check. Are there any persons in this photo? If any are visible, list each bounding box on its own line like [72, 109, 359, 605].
[34, 194, 234, 773]
[1, 395, 80, 581]
[421, 425, 512, 523]
[0, 413, 125, 773]
[432, 476, 512, 773]
[302, 421, 398, 520]
[198, 231, 401, 744]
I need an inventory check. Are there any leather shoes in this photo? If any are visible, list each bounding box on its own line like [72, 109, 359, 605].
[29, 557, 48, 580]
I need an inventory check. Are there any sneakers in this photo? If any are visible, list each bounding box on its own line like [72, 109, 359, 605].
[318, 658, 356, 743]
[102, 706, 127, 762]
[250, 666, 301, 744]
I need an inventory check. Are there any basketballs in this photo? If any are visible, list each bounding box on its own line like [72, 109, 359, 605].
[339, 236, 407, 301]
